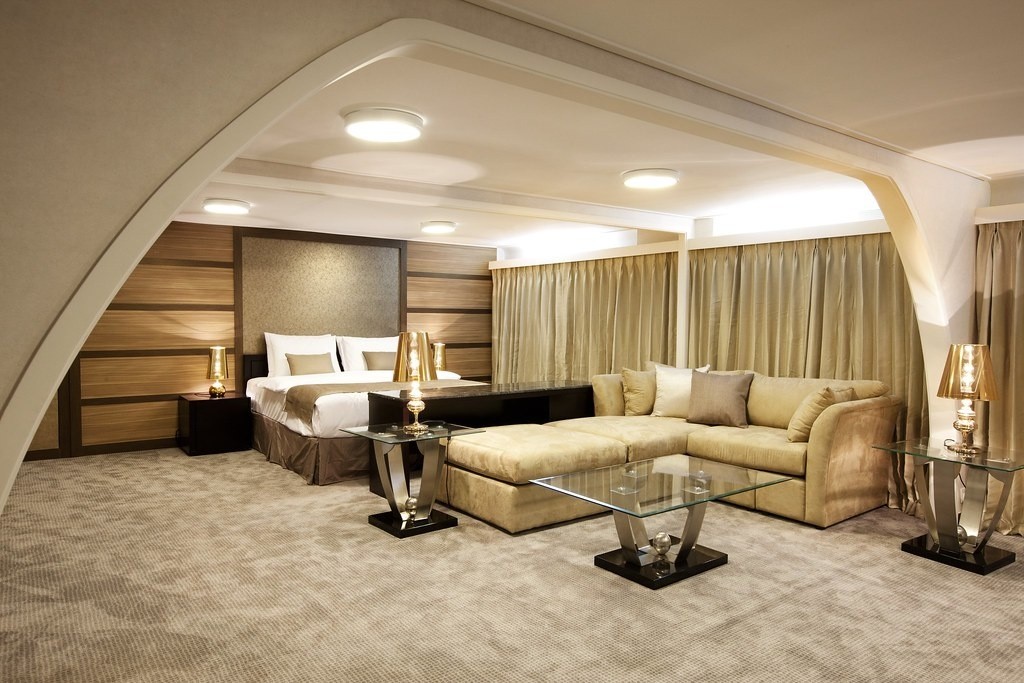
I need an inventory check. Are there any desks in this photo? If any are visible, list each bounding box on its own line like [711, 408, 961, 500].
[339, 419, 486, 539]
[369, 379, 592, 503]
[528, 453, 794, 591]
[872, 437, 1024, 576]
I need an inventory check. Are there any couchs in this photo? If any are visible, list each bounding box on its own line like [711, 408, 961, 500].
[436, 383, 903, 533]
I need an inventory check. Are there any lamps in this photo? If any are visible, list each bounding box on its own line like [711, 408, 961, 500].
[203, 199, 249, 216]
[431, 343, 447, 370]
[392, 330, 439, 431]
[936, 342, 998, 454]
[344, 110, 423, 143]
[206, 346, 230, 397]
[421, 221, 455, 234]
[622, 169, 680, 191]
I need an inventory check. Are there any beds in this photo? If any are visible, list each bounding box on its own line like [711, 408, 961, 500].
[242, 351, 461, 485]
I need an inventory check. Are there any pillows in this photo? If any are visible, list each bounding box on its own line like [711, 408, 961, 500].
[788, 387, 854, 442]
[621, 365, 754, 429]
[264, 331, 399, 377]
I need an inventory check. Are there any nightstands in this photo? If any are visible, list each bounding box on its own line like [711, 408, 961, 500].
[177, 392, 252, 459]
